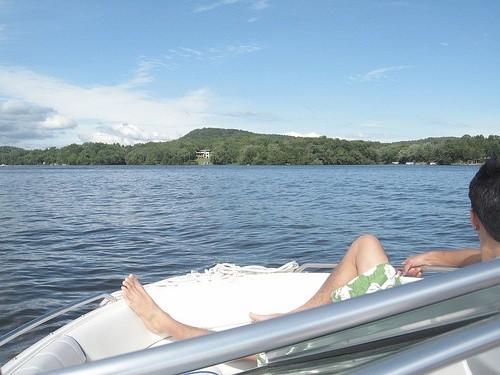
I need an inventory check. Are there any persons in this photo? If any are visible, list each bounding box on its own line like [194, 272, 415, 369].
[122, 155, 500, 367]
